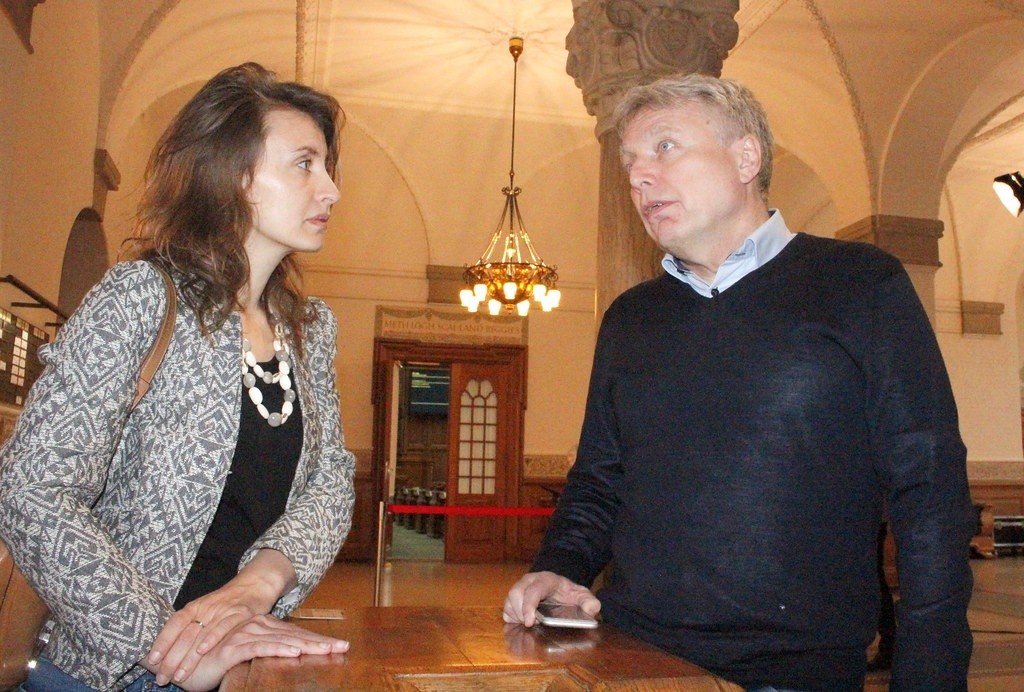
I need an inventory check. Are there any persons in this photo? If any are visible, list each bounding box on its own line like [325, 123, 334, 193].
[504, 74, 976, 692]
[0, 62, 357, 692]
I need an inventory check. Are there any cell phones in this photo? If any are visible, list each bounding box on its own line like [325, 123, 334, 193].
[535, 602, 598, 628]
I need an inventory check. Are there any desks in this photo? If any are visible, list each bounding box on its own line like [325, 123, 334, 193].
[220, 606, 747, 692]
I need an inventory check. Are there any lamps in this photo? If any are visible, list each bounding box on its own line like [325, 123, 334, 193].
[993, 171, 1024, 218]
[458, 36, 562, 319]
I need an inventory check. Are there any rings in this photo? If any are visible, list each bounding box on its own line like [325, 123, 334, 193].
[190, 620, 205, 628]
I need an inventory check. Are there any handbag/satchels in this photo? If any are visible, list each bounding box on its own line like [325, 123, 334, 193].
[0, 542, 50, 689]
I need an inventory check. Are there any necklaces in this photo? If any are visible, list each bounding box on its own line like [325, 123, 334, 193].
[241, 293, 296, 427]
